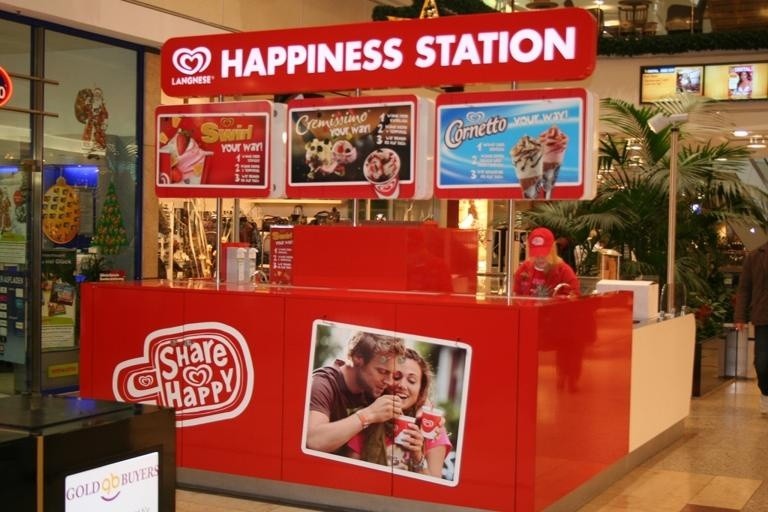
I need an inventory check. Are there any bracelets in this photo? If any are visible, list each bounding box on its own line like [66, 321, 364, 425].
[409, 455, 428, 467]
[355, 412, 368, 431]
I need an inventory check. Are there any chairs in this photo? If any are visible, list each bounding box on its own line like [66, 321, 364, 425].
[616, 0, 658, 41]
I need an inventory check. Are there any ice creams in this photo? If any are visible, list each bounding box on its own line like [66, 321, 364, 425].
[537, 127, 569, 200]
[509, 135, 544, 200]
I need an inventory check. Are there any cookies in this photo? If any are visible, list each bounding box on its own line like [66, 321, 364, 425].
[42, 184, 81, 244]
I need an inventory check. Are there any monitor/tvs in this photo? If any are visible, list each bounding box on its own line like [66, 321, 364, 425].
[638, 64, 703, 106]
[703, 60, 767, 101]
[60, 162, 99, 189]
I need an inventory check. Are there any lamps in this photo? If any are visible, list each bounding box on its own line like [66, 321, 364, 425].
[647, 109, 691, 134]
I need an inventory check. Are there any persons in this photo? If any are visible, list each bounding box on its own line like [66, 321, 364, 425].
[302, 331, 408, 453]
[380, 348, 454, 477]
[732, 71, 753, 97]
[512, 226, 578, 301]
[735, 223, 768, 414]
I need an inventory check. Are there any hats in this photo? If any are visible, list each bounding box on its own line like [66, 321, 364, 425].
[528, 227, 554, 257]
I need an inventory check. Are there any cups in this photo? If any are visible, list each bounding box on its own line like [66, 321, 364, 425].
[419, 404, 443, 440]
[362, 146, 402, 199]
[393, 414, 417, 448]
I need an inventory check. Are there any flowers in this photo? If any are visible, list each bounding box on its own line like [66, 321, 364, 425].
[686, 297, 727, 339]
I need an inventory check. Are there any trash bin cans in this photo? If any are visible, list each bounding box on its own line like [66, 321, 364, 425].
[725, 327, 748, 378]
